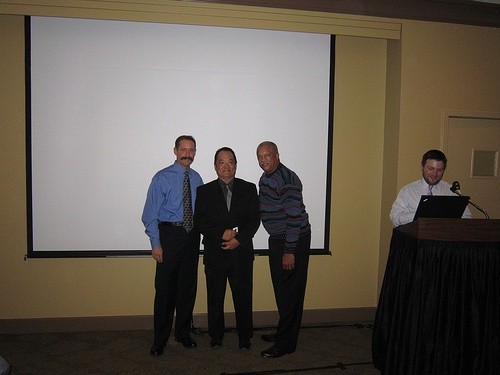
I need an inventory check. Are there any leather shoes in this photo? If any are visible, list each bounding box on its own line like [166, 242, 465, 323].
[175, 335, 197, 349]
[261, 344, 296, 359]
[261, 333, 277, 342]
[151, 342, 167, 357]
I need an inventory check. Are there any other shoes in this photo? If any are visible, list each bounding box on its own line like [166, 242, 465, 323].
[238, 338, 251, 351]
[210, 337, 222, 349]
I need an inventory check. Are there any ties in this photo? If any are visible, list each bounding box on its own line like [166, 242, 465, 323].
[182, 171, 195, 234]
[225, 184, 233, 212]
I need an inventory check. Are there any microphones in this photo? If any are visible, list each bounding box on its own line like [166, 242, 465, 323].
[451, 181, 459, 192]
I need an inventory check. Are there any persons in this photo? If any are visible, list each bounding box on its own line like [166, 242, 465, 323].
[257, 141, 311, 358]
[193, 147, 261, 351]
[142, 135, 204, 357]
[390, 150, 472, 227]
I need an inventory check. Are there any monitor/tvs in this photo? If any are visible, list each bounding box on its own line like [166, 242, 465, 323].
[413, 196, 470, 221]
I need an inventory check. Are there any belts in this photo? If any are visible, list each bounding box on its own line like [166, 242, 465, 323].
[161, 222, 182, 227]
